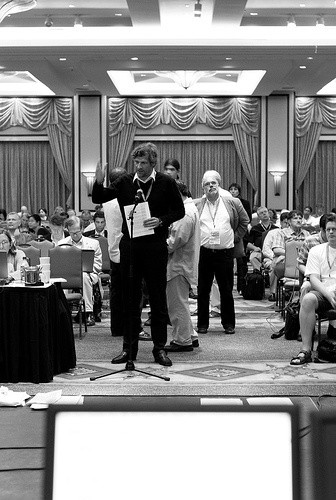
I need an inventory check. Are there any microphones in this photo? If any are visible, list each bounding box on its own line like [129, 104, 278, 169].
[134, 188, 144, 202]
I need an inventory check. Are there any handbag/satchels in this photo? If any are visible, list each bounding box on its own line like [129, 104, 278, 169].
[243, 269, 265, 300]
[284, 300, 317, 340]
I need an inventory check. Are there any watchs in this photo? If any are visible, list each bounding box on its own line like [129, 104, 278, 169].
[158, 217, 163, 226]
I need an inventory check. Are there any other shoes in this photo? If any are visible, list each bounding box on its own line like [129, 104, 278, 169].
[139, 330, 153, 341]
[209, 310, 221, 318]
[143, 318, 152, 326]
[170, 338, 200, 348]
[225, 327, 235, 334]
[111, 352, 127, 364]
[126, 360, 135, 370]
[189, 291, 196, 299]
[85, 314, 94, 326]
[190, 308, 198, 316]
[168, 342, 193, 352]
[197, 328, 207, 334]
[154, 354, 172, 366]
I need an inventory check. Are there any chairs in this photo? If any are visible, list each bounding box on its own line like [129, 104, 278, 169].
[47, 242, 89, 340]
[260, 228, 271, 276]
[280, 235, 305, 320]
[88, 234, 112, 286]
[17, 243, 42, 267]
[27, 238, 55, 257]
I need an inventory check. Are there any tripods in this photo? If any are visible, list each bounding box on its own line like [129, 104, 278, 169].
[90, 199, 170, 382]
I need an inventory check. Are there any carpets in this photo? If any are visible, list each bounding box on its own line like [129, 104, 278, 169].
[0, 282, 336, 399]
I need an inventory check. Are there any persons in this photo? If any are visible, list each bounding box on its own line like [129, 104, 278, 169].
[0, 158, 222, 326]
[227, 184, 252, 295]
[164, 183, 201, 352]
[91, 142, 185, 368]
[247, 205, 336, 365]
[194, 170, 250, 334]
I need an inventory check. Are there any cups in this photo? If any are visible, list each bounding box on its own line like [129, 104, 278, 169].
[38, 256, 50, 284]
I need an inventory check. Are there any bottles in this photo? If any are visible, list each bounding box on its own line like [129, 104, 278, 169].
[20, 257, 29, 283]
[25, 266, 39, 286]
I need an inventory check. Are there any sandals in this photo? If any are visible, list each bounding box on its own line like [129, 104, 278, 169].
[290, 350, 313, 365]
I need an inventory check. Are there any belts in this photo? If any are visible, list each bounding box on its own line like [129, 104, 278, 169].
[201, 247, 232, 252]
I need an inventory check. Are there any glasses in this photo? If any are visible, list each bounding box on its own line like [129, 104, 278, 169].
[69, 229, 82, 236]
[325, 229, 336, 231]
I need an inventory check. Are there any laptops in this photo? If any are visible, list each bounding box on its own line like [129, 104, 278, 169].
[82, 250, 95, 272]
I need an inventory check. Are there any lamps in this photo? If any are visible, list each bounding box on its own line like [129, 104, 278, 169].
[269, 170, 287, 197]
[82, 171, 96, 197]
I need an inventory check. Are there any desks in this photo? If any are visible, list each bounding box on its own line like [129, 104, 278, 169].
[0, 278, 78, 384]
